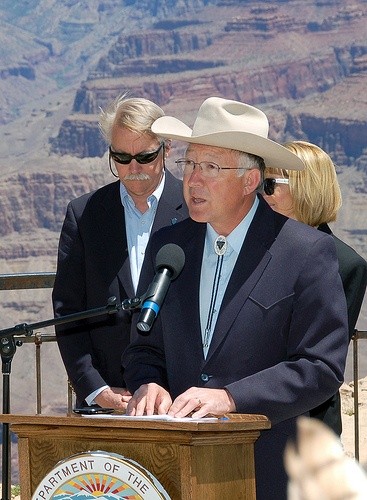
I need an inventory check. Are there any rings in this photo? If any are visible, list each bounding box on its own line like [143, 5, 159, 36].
[197, 399, 202, 406]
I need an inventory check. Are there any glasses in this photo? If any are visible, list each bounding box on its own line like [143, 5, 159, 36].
[264, 178, 288, 196]
[109, 143, 164, 165]
[174, 158, 252, 177]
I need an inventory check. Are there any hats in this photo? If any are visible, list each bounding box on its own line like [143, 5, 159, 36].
[149, 97, 305, 171]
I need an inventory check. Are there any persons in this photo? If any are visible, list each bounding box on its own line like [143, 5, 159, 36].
[264, 141, 367, 446]
[52, 97, 190, 416]
[120, 97, 348, 500]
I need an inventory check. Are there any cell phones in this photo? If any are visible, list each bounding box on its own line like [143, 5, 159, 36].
[72, 408, 115, 415]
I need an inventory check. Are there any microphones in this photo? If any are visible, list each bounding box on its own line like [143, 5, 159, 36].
[136, 243, 185, 332]
